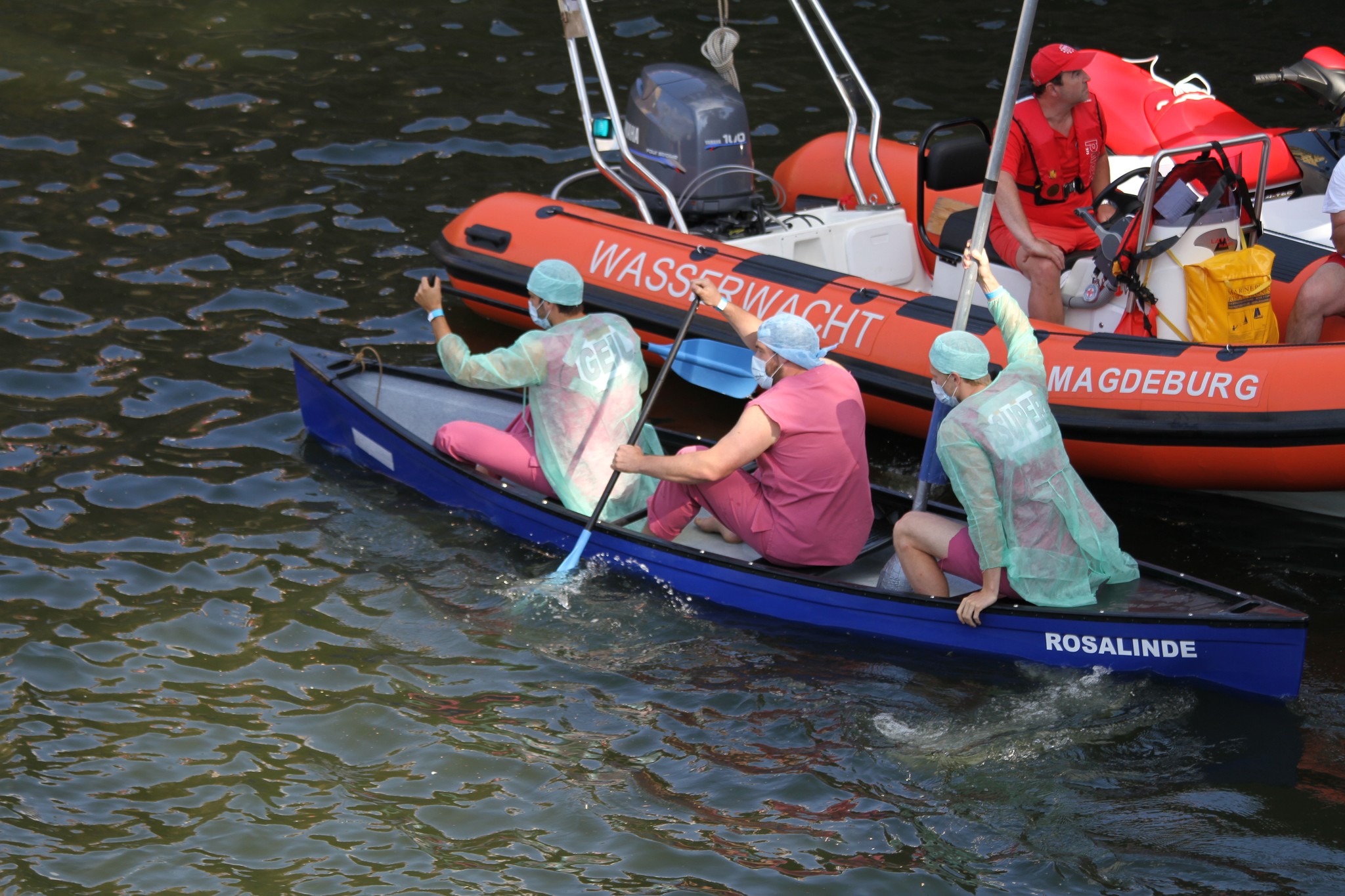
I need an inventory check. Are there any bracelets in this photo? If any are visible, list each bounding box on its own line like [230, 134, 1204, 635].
[1097, 198, 1115, 205]
[984, 288, 1003, 301]
[715, 295, 728, 311]
[428, 309, 445, 323]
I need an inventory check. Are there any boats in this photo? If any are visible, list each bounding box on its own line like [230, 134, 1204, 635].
[403, 0, 1345, 495]
[285, 346, 1312, 703]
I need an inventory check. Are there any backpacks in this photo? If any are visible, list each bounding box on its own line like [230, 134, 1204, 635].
[1143, 227, 1279, 343]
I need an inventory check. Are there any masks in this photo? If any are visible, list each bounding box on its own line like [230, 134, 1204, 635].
[528, 299, 553, 330]
[751, 353, 784, 389]
[931, 373, 960, 408]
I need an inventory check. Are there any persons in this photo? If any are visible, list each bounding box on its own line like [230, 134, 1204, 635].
[986, 43, 1125, 324]
[893, 239, 1140, 628]
[414, 259, 666, 523]
[1285, 150, 1345, 345]
[609, 278, 875, 573]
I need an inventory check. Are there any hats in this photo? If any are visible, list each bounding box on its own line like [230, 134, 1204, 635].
[1030, 44, 1097, 87]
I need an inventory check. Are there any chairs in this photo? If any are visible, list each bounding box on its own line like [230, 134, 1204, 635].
[917, 118, 1104, 333]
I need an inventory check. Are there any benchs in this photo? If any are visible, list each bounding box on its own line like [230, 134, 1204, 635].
[751, 518, 894, 576]
